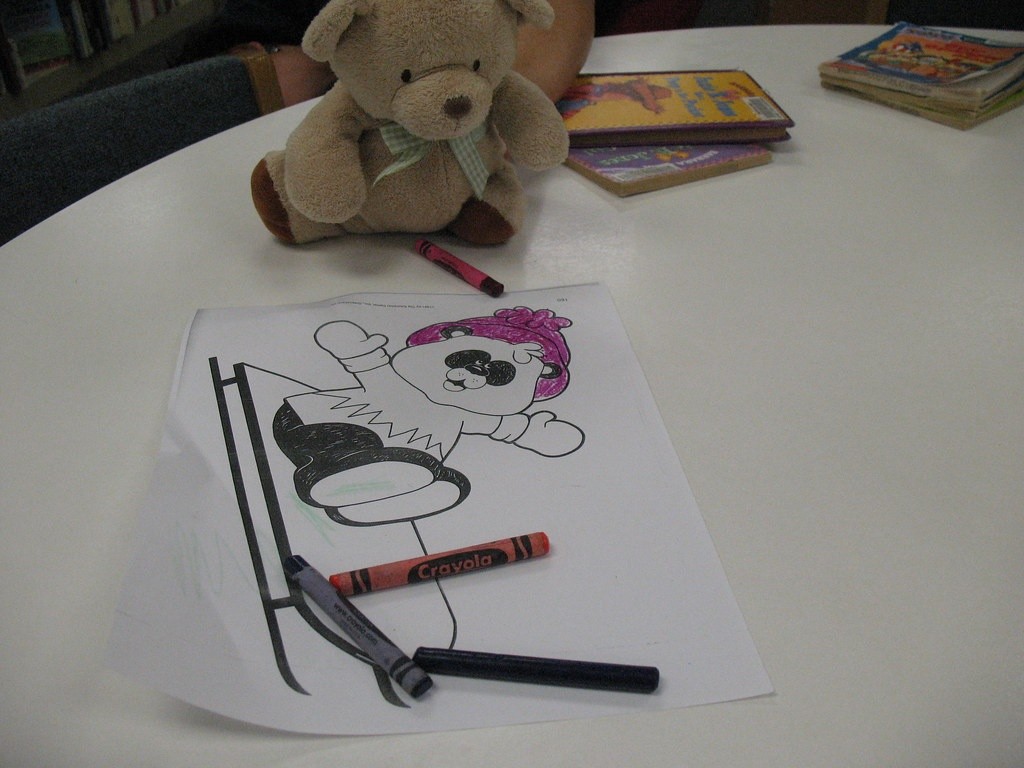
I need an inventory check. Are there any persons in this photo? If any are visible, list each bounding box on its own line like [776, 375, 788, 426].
[258, 1, 594, 110]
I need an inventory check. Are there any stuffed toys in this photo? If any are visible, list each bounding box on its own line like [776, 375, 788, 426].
[250, 1, 569, 248]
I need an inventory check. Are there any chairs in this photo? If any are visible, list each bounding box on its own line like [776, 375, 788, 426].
[0, 42, 285, 249]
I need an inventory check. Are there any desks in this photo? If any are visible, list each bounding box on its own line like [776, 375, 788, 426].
[0, 23, 1024, 767]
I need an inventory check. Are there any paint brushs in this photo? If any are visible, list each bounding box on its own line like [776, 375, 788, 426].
[331, 532, 551, 596]
[284, 554, 433, 700]
[413, 645, 660, 694]
[415, 238, 504, 298]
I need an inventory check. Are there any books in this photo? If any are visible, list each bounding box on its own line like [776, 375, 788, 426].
[561, 145, 773, 199]
[553, 68, 795, 150]
[818, 20, 1024, 130]
